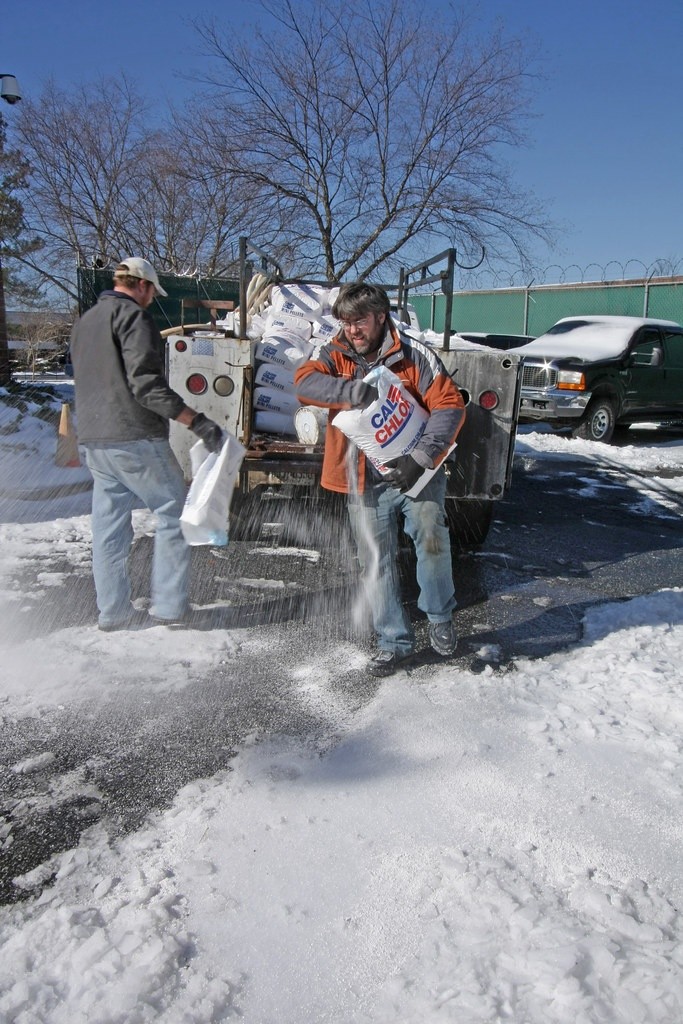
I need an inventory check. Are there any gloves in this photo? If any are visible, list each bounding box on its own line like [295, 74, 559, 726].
[187, 414, 223, 455]
[351, 378, 379, 411]
[382, 447, 434, 495]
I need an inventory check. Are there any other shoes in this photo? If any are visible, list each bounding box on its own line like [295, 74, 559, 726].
[151, 606, 209, 624]
[98, 608, 150, 631]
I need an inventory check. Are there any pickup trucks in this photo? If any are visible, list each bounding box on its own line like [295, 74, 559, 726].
[164, 233, 523, 549]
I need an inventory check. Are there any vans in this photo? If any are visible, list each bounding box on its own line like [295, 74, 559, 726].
[504, 315, 683, 443]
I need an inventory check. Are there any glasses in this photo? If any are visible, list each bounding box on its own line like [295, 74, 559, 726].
[339, 311, 375, 331]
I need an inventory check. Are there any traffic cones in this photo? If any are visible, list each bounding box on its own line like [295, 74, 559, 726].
[51, 403, 80, 467]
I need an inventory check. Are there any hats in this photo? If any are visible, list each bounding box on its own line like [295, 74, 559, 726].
[114, 258, 170, 297]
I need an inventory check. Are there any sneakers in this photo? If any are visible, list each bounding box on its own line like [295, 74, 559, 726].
[365, 647, 414, 676]
[429, 620, 457, 656]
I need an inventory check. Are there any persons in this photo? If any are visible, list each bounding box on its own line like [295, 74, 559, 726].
[294, 282, 467, 678]
[71, 256, 222, 631]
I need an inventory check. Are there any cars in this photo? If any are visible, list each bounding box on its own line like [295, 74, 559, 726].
[453, 330, 537, 351]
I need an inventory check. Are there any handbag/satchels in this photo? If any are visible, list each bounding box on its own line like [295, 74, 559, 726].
[332, 366, 459, 499]
[177, 430, 247, 546]
